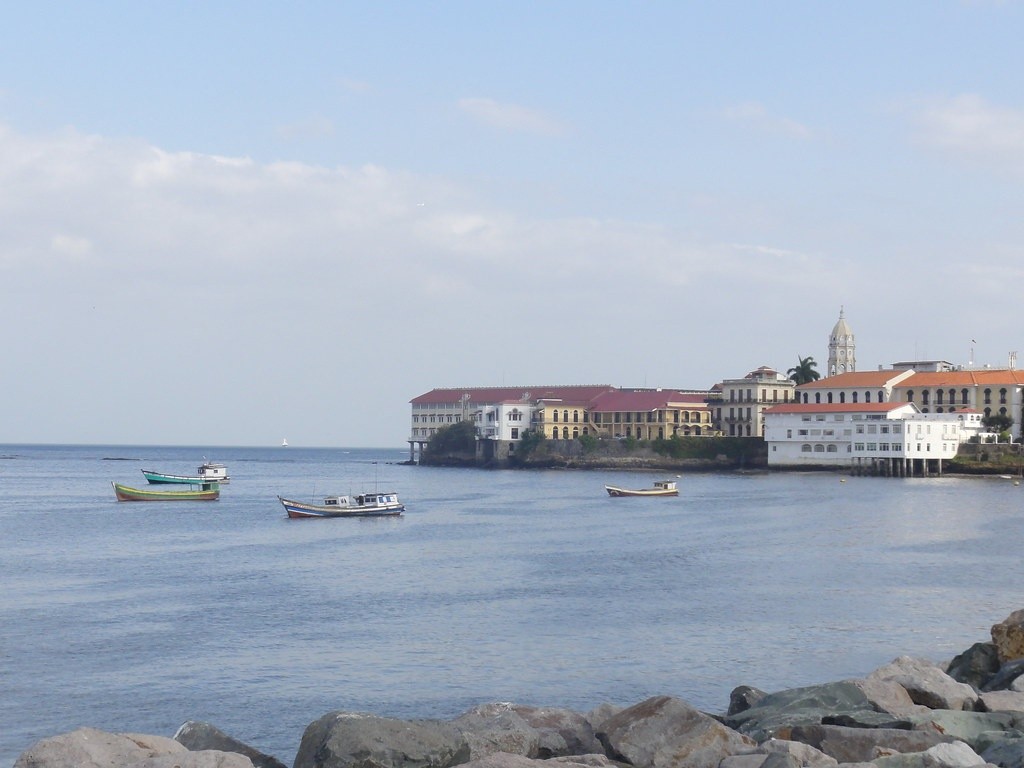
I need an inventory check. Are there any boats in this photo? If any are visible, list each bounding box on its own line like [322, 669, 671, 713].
[277, 489, 406, 519]
[141, 455, 230, 485]
[111, 481, 220, 501]
[605, 480, 679, 497]
